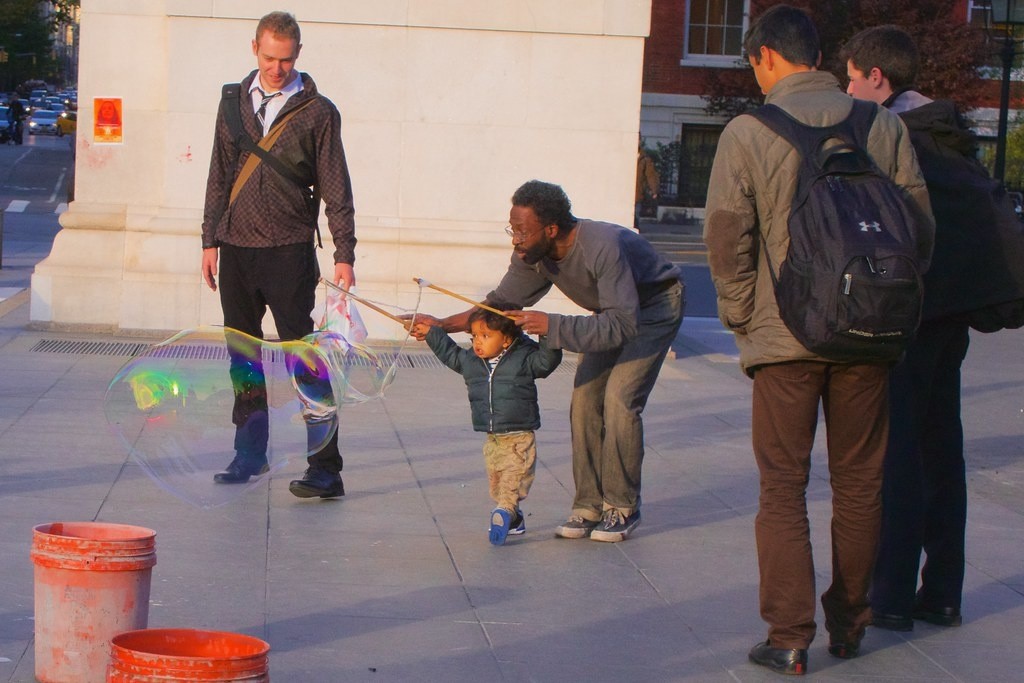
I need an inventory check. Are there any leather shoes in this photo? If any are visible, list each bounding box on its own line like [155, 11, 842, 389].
[289, 466, 346, 499]
[214, 451, 270, 482]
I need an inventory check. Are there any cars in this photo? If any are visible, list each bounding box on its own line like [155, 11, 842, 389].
[57, 110, 78, 137]
[1007, 191, 1024, 221]
[27, 109, 59, 136]
[0, 106, 17, 144]
[0, 84, 78, 119]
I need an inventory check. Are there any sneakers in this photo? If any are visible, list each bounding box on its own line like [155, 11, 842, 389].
[553, 516, 600, 538]
[590, 509, 642, 542]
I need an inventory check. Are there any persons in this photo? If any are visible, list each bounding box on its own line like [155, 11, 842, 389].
[6, 94, 24, 140]
[839, 24, 969, 632]
[703, 3, 936, 673]
[201, 11, 358, 499]
[634, 132, 659, 234]
[396, 181, 686, 541]
[414, 301, 562, 547]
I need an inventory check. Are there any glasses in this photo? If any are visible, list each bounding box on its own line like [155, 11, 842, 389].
[505, 224, 547, 242]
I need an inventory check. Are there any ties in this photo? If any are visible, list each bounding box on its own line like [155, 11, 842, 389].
[254, 87, 283, 137]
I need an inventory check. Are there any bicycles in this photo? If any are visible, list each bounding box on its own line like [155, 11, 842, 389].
[7, 115, 25, 145]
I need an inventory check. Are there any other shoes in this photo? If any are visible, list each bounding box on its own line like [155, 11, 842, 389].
[507, 510, 525, 535]
[828, 644, 858, 658]
[488, 507, 510, 545]
[748, 638, 807, 674]
[870, 612, 913, 631]
[913, 585, 962, 626]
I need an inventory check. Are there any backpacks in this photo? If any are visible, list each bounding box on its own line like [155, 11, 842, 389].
[747, 98, 923, 363]
[928, 153, 1024, 333]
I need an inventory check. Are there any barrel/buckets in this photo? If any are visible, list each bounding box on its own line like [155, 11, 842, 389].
[31, 520, 157, 683]
[105, 627, 272, 683]
